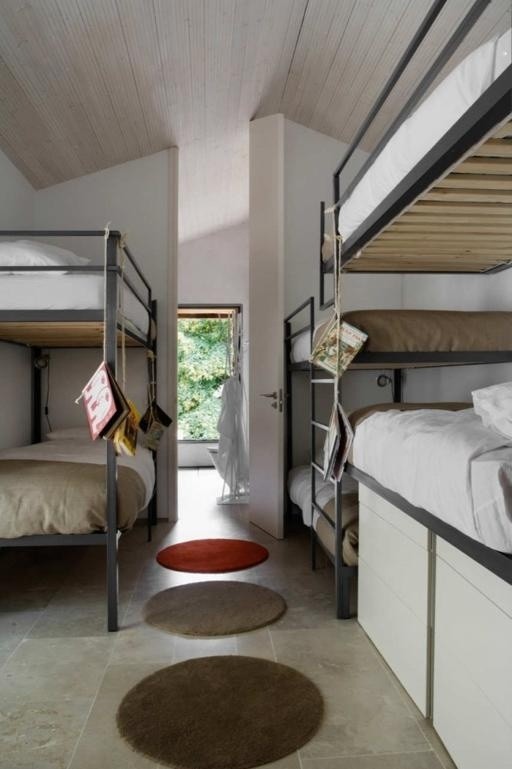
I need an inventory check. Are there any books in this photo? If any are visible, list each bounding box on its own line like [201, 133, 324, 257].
[114, 397, 140, 456]
[304, 319, 368, 379]
[323, 402, 353, 483]
[132, 400, 173, 452]
[78, 362, 133, 441]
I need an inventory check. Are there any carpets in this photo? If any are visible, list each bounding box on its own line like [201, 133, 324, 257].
[115, 655, 325, 769]
[157, 539, 269, 572]
[144, 581, 287, 636]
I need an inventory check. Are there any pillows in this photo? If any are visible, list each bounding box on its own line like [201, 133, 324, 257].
[45, 425, 103, 440]
[0, 239, 90, 276]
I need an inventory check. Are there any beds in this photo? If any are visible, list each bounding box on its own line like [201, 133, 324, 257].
[284, 0, 512, 620]
[1, 231, 157, 632]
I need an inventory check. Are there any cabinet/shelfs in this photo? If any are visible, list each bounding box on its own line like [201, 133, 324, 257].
[357, 482, 512, 768]
